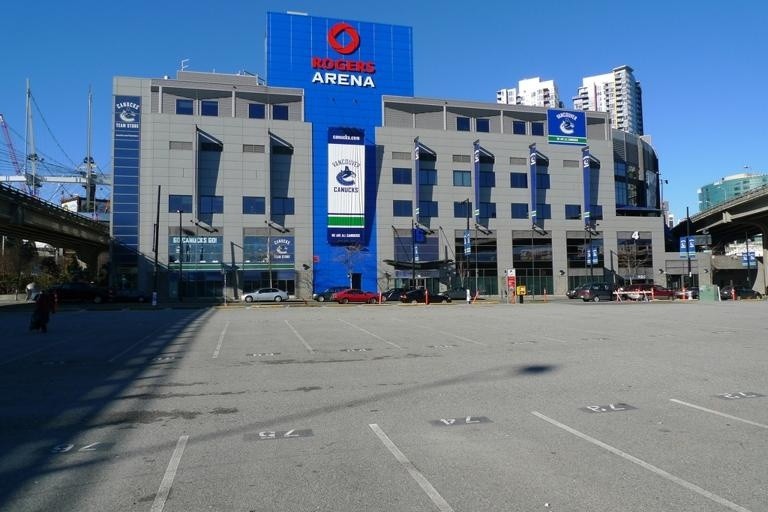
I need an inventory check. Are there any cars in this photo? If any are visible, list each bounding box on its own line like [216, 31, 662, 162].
[31, 282, 108, 304]
[719, 284, 762, 300]
[311, 287, 451, 304]
[565, 282, 698, 302]
[240, 288, 289, 303]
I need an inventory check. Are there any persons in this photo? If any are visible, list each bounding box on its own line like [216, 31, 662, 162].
[29, 288, 56, 334]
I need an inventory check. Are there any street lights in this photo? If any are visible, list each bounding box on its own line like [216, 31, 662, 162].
[220, 268, 227, 307]
[685, 206, 692, 302]
[717, 186, 727, 202]
[460, 198, 470, 306]
[744, 231, 751, 283]
[705, 200, 713, 208]
[176, 209, 182, 302]
[744, 166, 753, 190]
